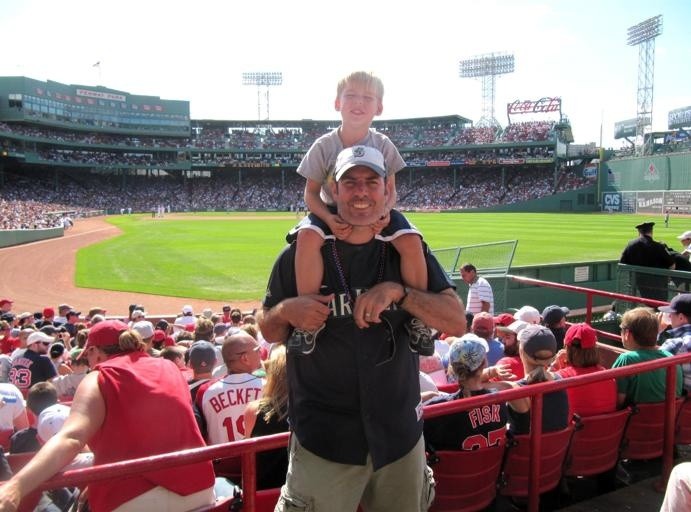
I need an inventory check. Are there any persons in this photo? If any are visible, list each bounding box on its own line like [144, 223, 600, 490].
[1, 113, 691, 298]
[656, 453, 691, 512]
[1, 299, 691, 510]
[0, 318, 220, 511]
[616, 219, 691, 299]
[459, 258, 499, 318]
[255, 142, 472, 511]
[281, 69, 438, 360]
[674, 226, 690, 266]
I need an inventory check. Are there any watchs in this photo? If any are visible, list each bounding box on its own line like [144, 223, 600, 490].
[396, 284, 411, 307]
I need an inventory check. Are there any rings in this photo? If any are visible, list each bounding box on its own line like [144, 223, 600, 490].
[365, 312, 371, 317]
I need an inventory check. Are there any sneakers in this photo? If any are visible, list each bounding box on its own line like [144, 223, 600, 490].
[404, 317, 434, 356]
[285, 323, 328, 359]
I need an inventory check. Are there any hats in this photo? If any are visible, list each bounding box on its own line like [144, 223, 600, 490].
[43, 307, 54, 317]
[154, 329, 165, 342]
[449, 335, 490, 372]
[36, 403, 70, 445]
[514, 306, 541, 325]
[183, 306, 192, 315]
[39, 325, 61, 335]
[657, 294, 691, 314]
[494, 313, 514, 326]
[488, 357, 524, 383]
[636, 221, 655, 231]
[676, 231, 691, 241]
[334, 144, 386, 183]
[472, 311, 494, 333]
[156, 319, 168, 328]
[132, 321, 155, 340]
[163, 336, 175, 346]
[564, 322, 596, 350]
[189, 340, 215, 370]
[25, 332, 55, 346]
[496, 319, 529, 337]
[18, 312, 33, 322]
[131, 309, 145, 318]
[516, 324, 558, 360]
[213, 323, 231, 333]
[0, 298, 13, 308]
[58, 304, 72, 310]
[541, 305, 569, 324]
[75, 321, 128, 361]
[65, 311, 81, 318]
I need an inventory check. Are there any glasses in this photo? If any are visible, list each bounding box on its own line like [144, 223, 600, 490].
[137, 316, 144, 318]
[618, 324, 630, 331]
[38, 341, 50, 346]
[236, 344, 261, 357]
